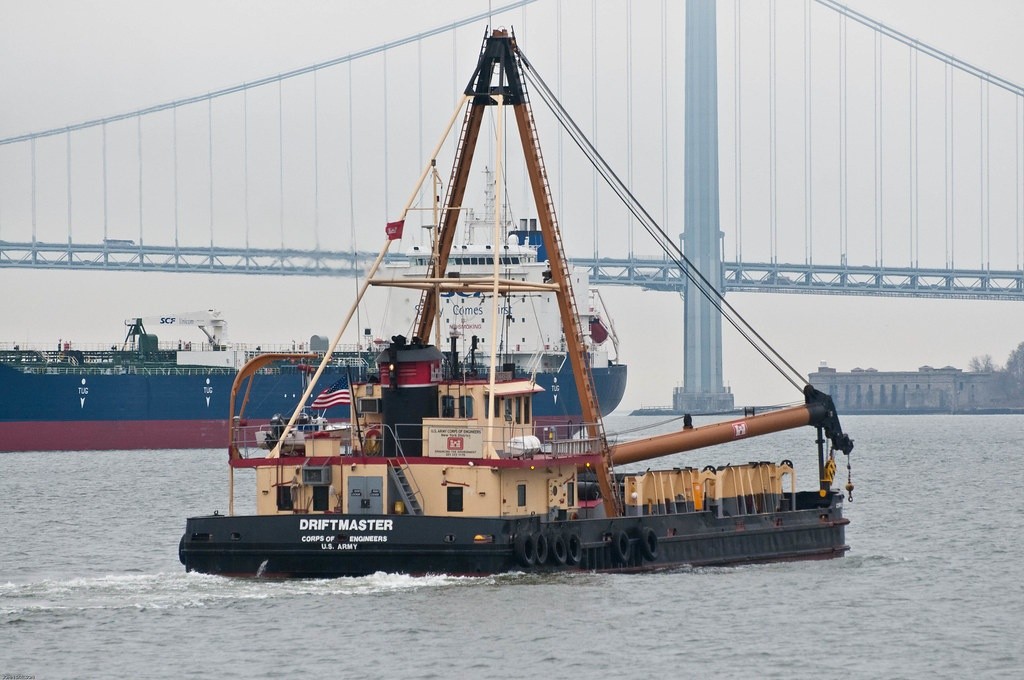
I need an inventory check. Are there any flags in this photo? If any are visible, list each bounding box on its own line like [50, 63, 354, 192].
[311, 374, 350, 410]
[386, 220, 404, 240]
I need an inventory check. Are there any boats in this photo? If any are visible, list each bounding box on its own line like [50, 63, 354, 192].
[180, 21, 858, 579]
[1, 313, 630, 454]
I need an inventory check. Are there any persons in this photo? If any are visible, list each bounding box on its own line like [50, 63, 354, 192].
[57, 340, 69, 351]
[179, 340, 191, 351]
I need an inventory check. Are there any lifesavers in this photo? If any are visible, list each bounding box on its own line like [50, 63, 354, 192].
[532, 531, 549, 566]
[639, 526, 660, 561]
[550, 534, 567, 565]
[364, 430, 382, 450]
[179, 534, 186, 565]
[612, 530, 631, 564]
[564, 532, 583, 566]
[514, 533, 536, 568]
